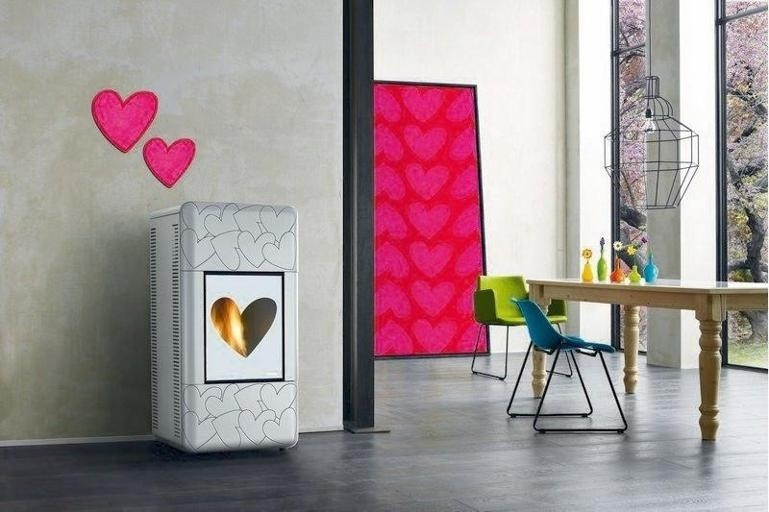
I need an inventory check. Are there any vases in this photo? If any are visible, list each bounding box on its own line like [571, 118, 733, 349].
[597, 255, 607, 281]
[610, 259, 625, 282]
[629, 265, 641, 283]
[582, 261, 593, 281]
[643, 254, 657, 283]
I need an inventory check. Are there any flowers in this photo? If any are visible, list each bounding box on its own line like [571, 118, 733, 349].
[627, 246, 638, 265]
[600, 236, 606, 258]
[581, 248, 593, 263]
[613, 241, 625, 258]
[642, 237, 654, 257]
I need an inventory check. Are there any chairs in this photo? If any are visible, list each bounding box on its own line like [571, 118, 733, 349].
[471, 275, 573, 380]
[506, 296, 628, 433]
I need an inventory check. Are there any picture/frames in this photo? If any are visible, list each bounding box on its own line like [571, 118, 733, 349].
[374, 80, 492, 360]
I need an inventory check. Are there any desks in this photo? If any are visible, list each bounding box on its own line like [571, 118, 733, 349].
[526, 279, 768, 441]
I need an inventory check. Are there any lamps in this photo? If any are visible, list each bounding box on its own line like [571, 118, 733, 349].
[604, 1, 699, 212]
